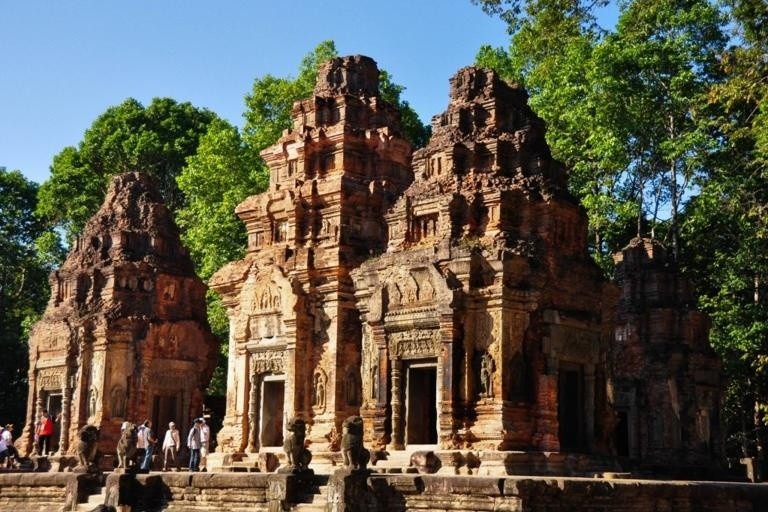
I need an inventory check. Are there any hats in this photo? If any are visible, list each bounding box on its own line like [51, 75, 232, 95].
[168, 416, 205, 427]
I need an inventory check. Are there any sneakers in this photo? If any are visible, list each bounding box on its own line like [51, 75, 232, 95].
[161, 466, 207, 472]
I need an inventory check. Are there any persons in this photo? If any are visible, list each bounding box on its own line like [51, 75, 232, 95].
[0, 426, 26, 465]
[2, 423, 15, 468]
[136, 424, 146, 463]
[199, 417, 211, 470]
[187, 418, 203, 471]
[161, 421, 182, 472]
[140, 418, 159, 471]
[35, 408, 55, 455]
